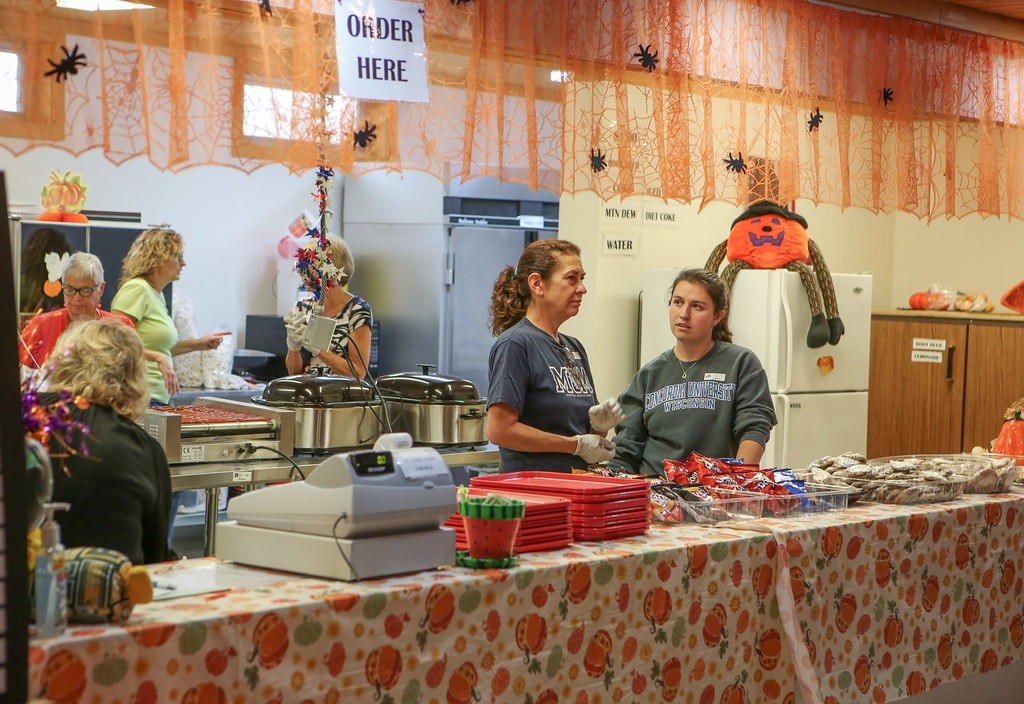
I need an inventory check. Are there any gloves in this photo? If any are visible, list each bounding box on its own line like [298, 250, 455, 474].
[573, 435, 617, 463]
[589, 398, 626, 433]
[283, 309, 321, 357]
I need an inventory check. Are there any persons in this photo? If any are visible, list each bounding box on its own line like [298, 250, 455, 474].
[609, 268, 777, 476]
[284, 235, 373, 381]
[19, 251, 135, 368]
[20, 227, 71, 313]
[111, 229, 233, 550]
[486, 239, 625, 473]
[46, 319, 178, 563]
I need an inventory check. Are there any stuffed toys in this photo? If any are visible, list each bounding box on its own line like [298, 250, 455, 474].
[706, 172, 844, 348]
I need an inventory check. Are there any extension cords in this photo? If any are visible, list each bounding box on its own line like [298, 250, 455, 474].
[302, 314, 337, 352]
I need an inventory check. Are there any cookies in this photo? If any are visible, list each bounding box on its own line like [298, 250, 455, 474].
[797, 446, 1024, 503]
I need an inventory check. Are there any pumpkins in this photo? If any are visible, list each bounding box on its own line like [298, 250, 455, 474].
[909, 289, 950, 310]
[990, 412, 1024, 467]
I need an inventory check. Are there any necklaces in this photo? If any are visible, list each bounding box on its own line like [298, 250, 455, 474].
[679, 358, 700, 378]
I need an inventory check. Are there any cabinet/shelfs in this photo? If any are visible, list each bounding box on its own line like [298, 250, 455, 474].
[867, 311, 1024, 460]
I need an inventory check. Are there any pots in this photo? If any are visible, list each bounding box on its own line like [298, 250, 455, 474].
[252, 366, 382, 455]
[375, 362, 488, 452]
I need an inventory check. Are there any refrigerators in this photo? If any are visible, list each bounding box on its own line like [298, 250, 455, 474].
[638, 269, 870, 469]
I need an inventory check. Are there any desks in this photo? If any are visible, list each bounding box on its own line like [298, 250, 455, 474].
[169, 446, 502, 557]
[29, 494, 1024, 704]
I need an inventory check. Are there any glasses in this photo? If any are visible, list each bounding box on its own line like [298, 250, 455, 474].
[62, 282, 99, 297]
[176, 255, 185, 262]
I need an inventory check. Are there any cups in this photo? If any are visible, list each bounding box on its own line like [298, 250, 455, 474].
[460, 498, 524, 559]
